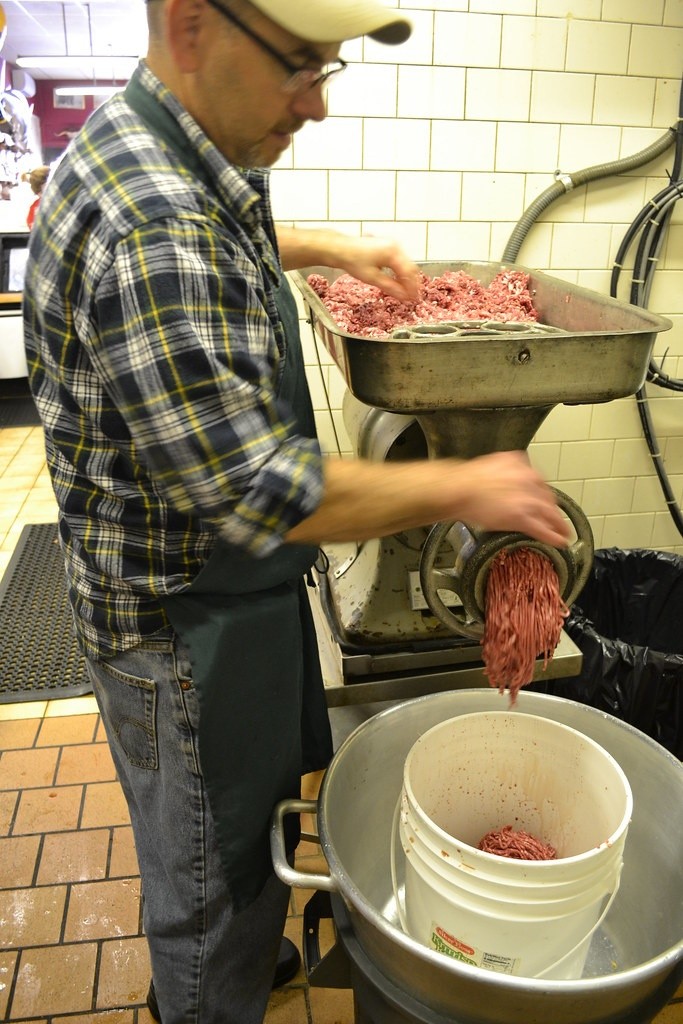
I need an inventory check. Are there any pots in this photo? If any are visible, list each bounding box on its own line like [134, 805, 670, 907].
[269, 687, 683, 1024]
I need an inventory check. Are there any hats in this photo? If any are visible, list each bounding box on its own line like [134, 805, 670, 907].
[251, 0, 413, 45]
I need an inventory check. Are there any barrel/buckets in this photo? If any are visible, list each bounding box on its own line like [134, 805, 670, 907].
[390, 711, 633, 980]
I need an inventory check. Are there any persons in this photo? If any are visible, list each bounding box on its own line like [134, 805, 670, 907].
[23, 0, 577, 1023]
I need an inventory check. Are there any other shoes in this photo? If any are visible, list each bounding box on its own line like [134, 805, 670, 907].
[146, 936, 301, 1024]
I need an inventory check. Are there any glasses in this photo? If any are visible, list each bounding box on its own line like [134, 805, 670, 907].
[205, 0, 347, 96]
